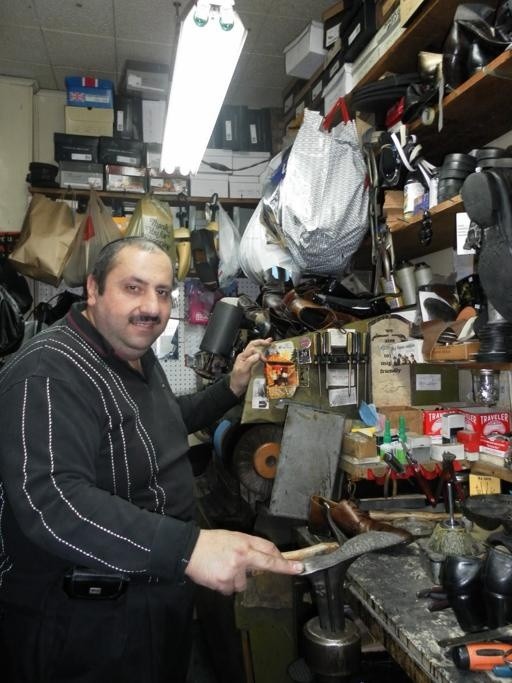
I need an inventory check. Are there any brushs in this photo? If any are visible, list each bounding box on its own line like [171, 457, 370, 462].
[247, 542, 339, 577]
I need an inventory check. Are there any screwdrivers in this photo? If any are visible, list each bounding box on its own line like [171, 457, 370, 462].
[322, 332, 331, 390]
[312, 332, 322, 399]
[346, 333, 355, 398]
[360, 332, 370, 403]
[353, 331, 362, 408]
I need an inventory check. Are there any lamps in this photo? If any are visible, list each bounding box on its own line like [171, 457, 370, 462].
[154, 0, 251, 182]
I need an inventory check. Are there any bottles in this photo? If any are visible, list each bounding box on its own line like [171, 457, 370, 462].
[379, 417, 393, 461]
[396, 415, 410, 462]
[379, 271, 406, 309]
[413, 262, 434, 289]
[403, 177, 426, 220]
[428, 166, 442, 211]
[393, 258, 420, 307]
[475, 369, 500, 408]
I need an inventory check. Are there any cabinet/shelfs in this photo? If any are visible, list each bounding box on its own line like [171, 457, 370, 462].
[320, 0, 512, 264]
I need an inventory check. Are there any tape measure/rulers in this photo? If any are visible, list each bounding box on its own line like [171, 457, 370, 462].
[437, 624, 512, 648]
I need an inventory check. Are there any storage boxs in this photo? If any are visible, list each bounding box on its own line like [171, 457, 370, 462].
[54, 58, 271, 199]
[280, 0, 425, 123]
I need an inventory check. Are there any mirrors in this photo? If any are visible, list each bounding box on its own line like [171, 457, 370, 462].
[151, 286, 179, 360]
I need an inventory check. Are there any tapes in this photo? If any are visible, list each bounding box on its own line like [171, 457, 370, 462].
[421, 108, 435, 126]
[430, 442, 465, 462]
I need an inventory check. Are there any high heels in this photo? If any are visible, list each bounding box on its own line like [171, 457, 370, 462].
[439, 552, 486, 632]
[478, 547, 512, 630]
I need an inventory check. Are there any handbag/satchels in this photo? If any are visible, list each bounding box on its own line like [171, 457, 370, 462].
[124, 200, 176, 268]
[277, 108, 373, 276]
[6, 191, 86, 288]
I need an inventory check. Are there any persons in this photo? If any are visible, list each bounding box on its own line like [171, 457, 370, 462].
[1, 235, 308, 680]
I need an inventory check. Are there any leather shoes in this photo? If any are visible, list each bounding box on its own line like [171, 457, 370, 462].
[461, 164, 512, 322]
[322, 279, 391, 321]
[307, 494, 415, 552]
[287, 297, 345, 332]
